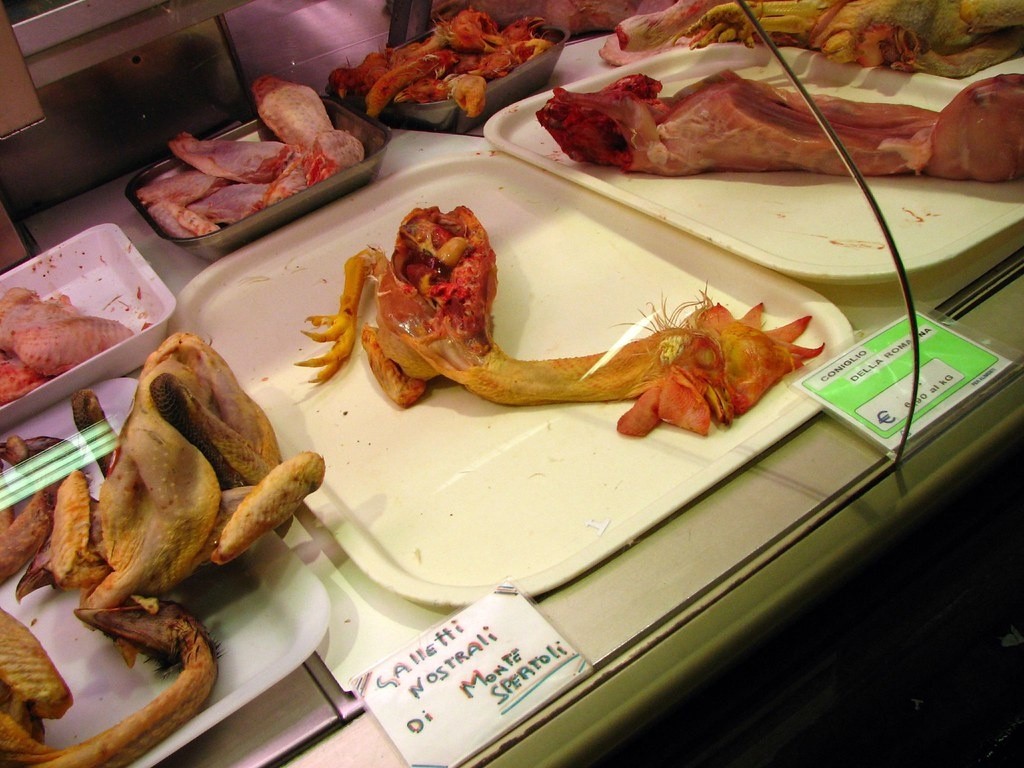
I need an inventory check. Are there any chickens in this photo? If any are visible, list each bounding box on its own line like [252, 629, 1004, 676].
[294, 205, 826, 438]
[0, 332, 324, 768]
[667, 0, 1024, 81]
